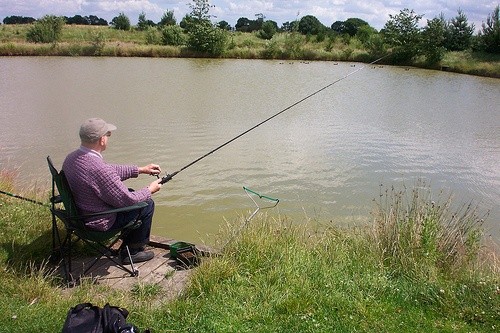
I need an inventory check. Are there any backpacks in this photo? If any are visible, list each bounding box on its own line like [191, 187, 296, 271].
[61, 302, 139, 333]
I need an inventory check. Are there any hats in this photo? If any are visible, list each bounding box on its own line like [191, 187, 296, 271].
[79, 118, 116, 142]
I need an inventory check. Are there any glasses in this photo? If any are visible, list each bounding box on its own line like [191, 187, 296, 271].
[104, 131, 111, 137]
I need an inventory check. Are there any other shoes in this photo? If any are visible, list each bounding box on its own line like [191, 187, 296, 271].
[120, 246, 154, 264]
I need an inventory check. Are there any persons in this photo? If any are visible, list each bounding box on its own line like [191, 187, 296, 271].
[60, 117, 163, 266]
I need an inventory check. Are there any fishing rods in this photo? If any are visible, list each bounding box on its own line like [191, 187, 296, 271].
[153, 51, 403, 185]
